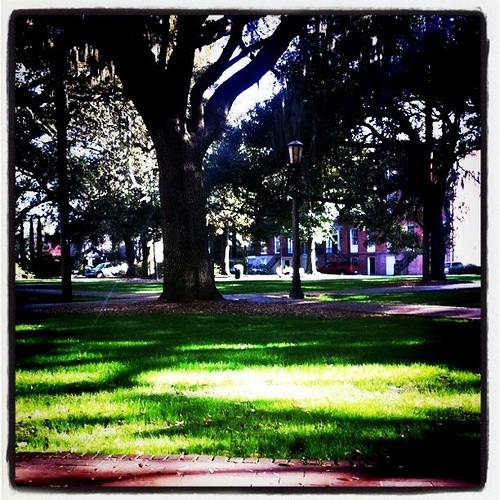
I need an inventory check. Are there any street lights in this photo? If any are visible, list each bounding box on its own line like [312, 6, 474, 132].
[285, 140, 304, 300]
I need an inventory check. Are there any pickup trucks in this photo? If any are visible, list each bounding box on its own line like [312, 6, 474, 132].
[320, 261, 361, 275]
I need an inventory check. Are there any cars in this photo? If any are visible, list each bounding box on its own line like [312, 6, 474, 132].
[444, 261, 463, 274]
[86, 261, 128, 278]
